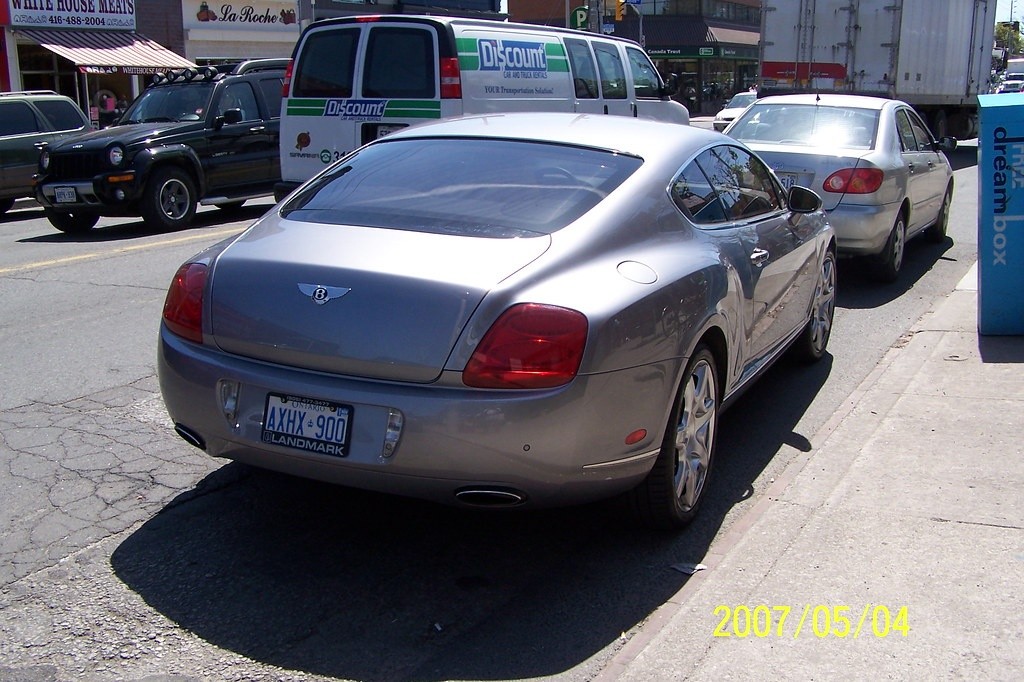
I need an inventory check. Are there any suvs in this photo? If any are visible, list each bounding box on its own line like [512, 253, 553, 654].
[1, 89, 96, 218]
[30, 57, 292, 234]
[998, 72, 1024, 93]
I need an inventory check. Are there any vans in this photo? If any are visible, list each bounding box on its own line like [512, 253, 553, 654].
[273, 14, 691, 205]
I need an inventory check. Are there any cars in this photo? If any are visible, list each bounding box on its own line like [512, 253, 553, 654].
[156, 111, 838, 533]
[720, 94, 959, 284]
[713, 92, 758, 132]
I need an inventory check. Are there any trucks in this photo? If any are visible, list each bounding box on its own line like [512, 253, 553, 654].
[760, 0, 1008, 162]
